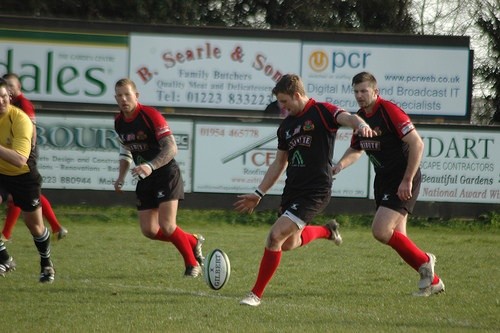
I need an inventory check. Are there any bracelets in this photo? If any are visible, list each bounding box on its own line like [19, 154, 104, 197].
[146, 162, 155, 171]
[253, 188, 265, 199]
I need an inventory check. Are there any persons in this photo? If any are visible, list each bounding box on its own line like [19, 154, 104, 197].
[261, 87, 289, 125]
[234, 74, 378, 306]
[0, 78, 56, 285]
[0, 73, 68, 244]
[330, 71, 446, 296]
[112, 78, 206, 279]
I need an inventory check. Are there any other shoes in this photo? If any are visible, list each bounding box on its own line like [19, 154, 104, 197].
[326, 219, 343, 246]
[58, 227, 67, 239]
[193, 233, 206, 264]
[412, 278, 445, 298]
[0, 256, 16, 277]
[416, 252, 436, 289]
[183, 260, 203, 279]
[39, 260, 55, 285]
[239, 292, 263, 307]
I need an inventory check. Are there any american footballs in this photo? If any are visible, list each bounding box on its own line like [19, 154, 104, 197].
[204, 248, 231, 292]
